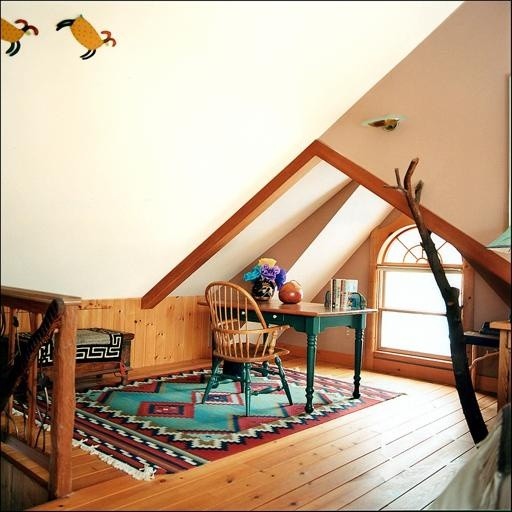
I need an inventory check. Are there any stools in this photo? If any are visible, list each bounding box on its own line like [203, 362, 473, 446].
[0, 327, 135, 398]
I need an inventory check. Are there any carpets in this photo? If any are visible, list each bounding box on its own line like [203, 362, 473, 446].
[8, 361, 406, 482]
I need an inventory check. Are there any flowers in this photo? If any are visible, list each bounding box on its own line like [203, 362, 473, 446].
[243, 257, 286, 292]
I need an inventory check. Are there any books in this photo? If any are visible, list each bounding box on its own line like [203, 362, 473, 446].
[340, 278, 359, 310]
[330, 278, 344, 310]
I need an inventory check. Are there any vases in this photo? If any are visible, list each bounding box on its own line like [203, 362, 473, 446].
[251, 279, 275, 301]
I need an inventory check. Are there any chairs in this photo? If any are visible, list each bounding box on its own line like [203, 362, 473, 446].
[198, 281, 293, 418]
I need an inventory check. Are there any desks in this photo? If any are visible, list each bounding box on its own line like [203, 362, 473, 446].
[198, 295, 379, 414]
